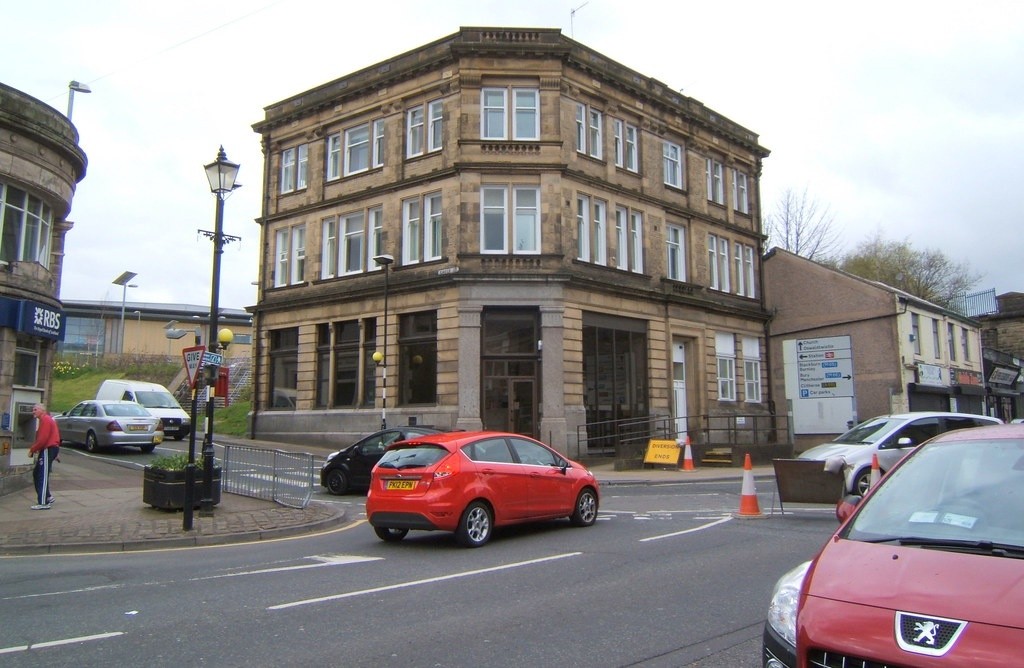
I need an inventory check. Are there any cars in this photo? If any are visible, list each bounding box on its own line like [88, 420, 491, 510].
[797, 410, 1005, 504]
[52, 399, 164, 455]
[761, 423, 1024, 668]
[320, 425, 487, 497]
[365, 430, 602, 549]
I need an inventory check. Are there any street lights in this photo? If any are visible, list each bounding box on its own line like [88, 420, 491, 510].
[111, 270, 139, 353]
[66, 81, 93, 124]
[373, 254, 394, 428]
[165, 323, 200, 530]
[133, 310, 141, 354]
[199, 144, 242, 518]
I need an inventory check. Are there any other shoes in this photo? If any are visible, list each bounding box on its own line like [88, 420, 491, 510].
[31, 505, 50, 509]
[47, 497, 55, 502]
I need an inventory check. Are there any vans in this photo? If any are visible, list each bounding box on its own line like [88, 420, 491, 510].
[96, 378, 192, 441]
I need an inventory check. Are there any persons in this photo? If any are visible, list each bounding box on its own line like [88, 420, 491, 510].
[27, 403, 60, 510]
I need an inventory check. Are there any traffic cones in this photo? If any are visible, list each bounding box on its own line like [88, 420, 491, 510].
[679, 436, 697, 471]
[867, 452, 882, 491]
[732, 454, 769, 520]
